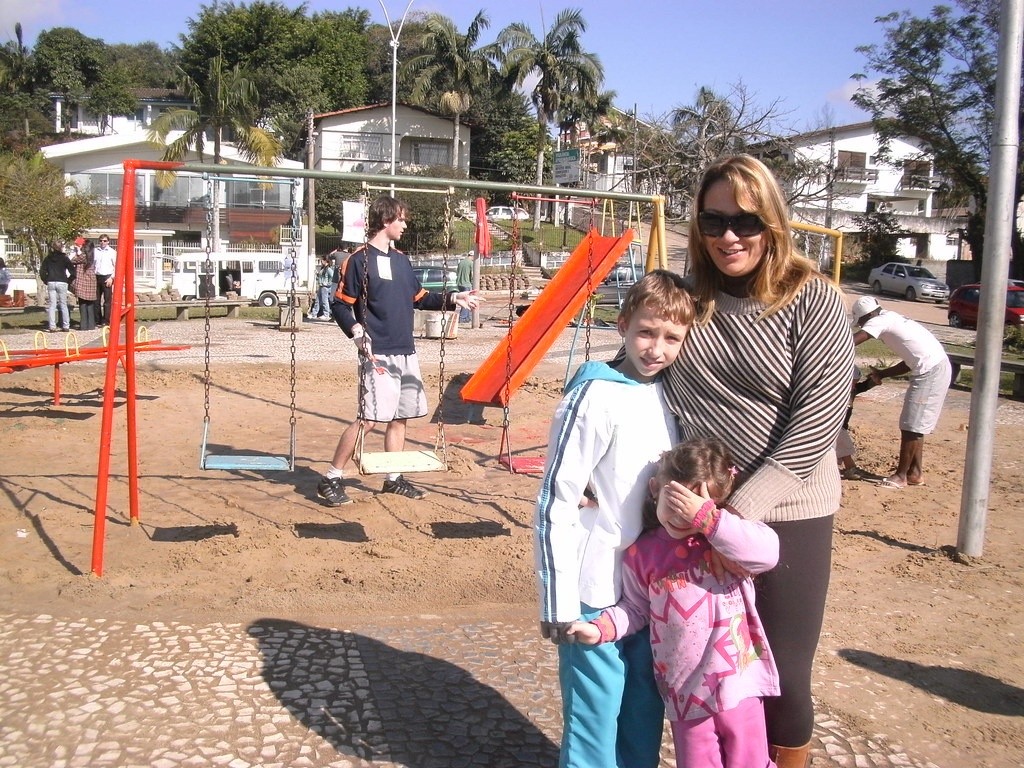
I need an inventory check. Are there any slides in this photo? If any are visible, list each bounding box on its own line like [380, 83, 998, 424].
[457, 226, 635, 407]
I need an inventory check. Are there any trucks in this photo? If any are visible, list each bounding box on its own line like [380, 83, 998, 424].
[153, 253, 296, 308]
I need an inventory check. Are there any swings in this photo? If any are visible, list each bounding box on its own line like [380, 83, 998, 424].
[349, 180, 458, 478]
[497, 189, 600, 476]
[195, 172, 301, 473]
[801, 230, 827, 272]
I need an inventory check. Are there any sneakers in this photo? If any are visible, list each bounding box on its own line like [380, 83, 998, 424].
[316, 476, 354, 507]
[382, 475, 427, 500]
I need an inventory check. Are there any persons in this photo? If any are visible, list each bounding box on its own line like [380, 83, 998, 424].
[534, 270, 696, 768]
[316, 195, 486, 509]
[835, 364, 883, 479]
[456, 249, 474, 322]
[850, 295, 953, 490]
[659, 153, 855, 768]
[306, 243, 352, 321]
[39, 229, 117, 333]
[565, 436, 782, 768]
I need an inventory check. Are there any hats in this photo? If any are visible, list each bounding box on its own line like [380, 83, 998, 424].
[853, 365, 862, 379]
[74, 237, 85, 245]
[468, 250, 475, 256]
[850, 295, 881, 328]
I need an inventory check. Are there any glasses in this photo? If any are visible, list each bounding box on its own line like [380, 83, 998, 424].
[698, 211, 766, 237]
[100, 240, 108, 242]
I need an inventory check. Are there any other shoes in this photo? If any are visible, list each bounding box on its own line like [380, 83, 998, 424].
[843, 467, 864, 479]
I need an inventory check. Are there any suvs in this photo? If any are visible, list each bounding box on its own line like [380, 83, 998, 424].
[412, 265, 459, 296]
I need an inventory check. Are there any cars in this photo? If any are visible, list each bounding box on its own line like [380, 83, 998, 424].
[976, 279, 1024, 286]
[868, 262, 951, 302]
[485, 206, 530, 219]
[947, 284, 1024, 331]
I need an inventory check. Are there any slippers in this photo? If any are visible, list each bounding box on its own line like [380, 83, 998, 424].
[875, 477, 904, 489]
[908, 481, 924, 486]
